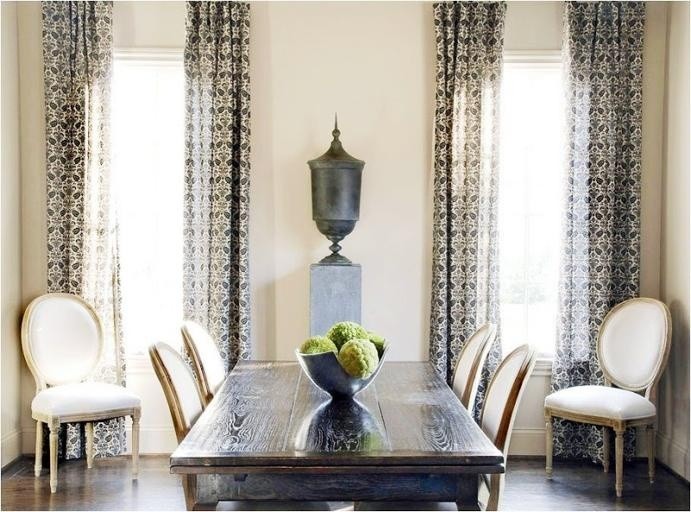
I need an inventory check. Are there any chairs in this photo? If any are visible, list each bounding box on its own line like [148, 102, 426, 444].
[544, 297, 673, 498]
[147, 338, 330, 511]
[451, 323, 498, 414]
[178, 318, 227, 411]
[21, 292, 142, 493]
[353, 342, 538, 511]
